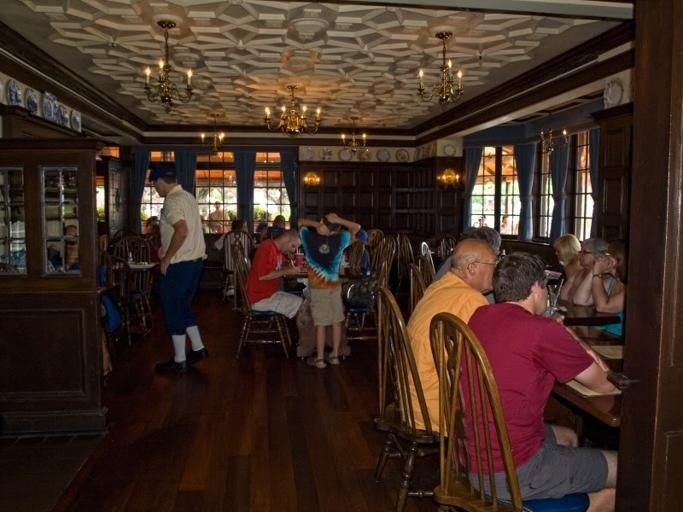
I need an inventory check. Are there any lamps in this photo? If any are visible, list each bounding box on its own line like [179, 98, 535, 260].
[262, 84, 323, 136]
[417, 31, 466, 106]
[538, 108, 569, 156]
[198, 113, 226, 154]
[341, 116, 369, 155]
[142, 17, 195, 113]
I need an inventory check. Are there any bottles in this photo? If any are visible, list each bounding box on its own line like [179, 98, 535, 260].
[500, 248, 506, 260]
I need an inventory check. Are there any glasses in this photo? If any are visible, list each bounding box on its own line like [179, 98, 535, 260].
[599, 251, 612, 257]
[474, 260, 499, 268]
[576, 251, 590, 256]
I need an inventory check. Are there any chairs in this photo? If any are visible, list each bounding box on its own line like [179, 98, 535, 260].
[223, 229, 623, 363]
[429, 311, 591, 512]
[374, 286, 449, 511]
[99, 227, 160, 378]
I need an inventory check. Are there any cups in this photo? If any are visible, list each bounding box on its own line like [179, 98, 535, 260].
[548, 284, 561, 306]
[293, 253, 305, 268]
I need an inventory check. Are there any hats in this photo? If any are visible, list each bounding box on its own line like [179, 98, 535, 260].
[148, 161, 177, 182]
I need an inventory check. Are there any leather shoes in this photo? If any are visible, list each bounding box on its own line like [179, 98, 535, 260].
[186, 347, 209, 366]
[155, 357, 189, 375]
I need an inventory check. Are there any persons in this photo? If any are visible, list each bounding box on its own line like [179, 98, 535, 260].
[148, 161, 208, 373]
[397, 238, 499, 439]
[433, 226, 625, 338]
[460, 252, 619, 501]
[136, 214, 158, 235]
[200, 201, 369, 368]
[70, 244, 120, 376]
[65, 225, 77, 236]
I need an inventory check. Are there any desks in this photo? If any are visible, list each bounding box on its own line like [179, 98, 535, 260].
[551, 324, 623, 429]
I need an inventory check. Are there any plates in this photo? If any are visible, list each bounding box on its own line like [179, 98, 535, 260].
[299, 142, 457, 163]
[24, 86, 38, 115]
[69, 108, 81, 130]
[604, 78, 624, 108]
[42, 91, 58, 123]
[5, 78, 23, 107]
[55, 103, 69, 126]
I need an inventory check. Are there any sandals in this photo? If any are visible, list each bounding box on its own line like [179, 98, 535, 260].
[306, 357, 329, 370]
[325, 352, 341, 366]
[102, 351, 113, 376]
